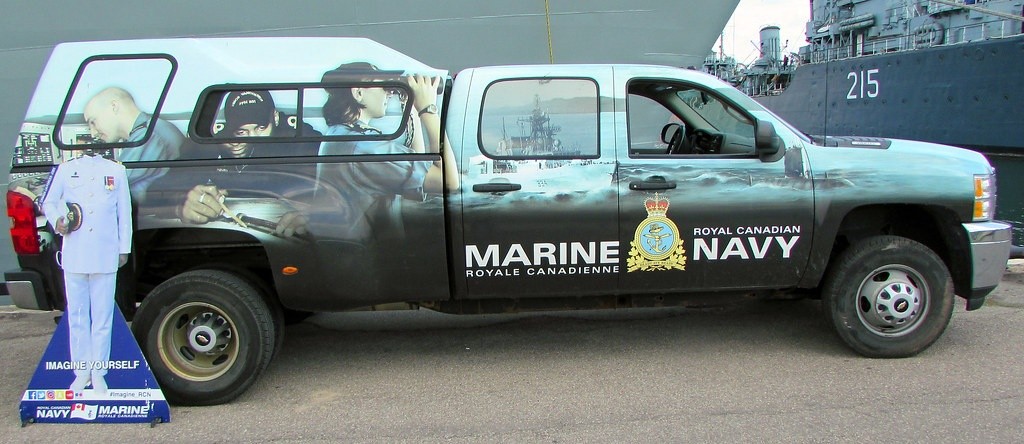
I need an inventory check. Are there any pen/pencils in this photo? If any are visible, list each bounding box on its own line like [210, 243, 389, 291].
[219, 203, 248, 229]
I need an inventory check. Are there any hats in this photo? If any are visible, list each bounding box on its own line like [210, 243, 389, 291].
[225, 90, 275, 129]
[56, 200, 83, 234]
[333, 62, 405, 80]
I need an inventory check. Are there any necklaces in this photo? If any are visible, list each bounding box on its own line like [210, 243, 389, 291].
[236, 147, 255, 174]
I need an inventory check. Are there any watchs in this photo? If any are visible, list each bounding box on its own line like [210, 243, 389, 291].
[418, 104, 439, 117]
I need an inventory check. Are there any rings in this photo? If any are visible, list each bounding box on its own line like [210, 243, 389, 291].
[199, 192, 206, 202]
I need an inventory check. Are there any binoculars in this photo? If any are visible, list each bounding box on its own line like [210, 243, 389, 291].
[388, 75, 445, 96]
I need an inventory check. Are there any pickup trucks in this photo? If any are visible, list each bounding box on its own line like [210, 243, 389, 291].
[3, 35, 1014, 407]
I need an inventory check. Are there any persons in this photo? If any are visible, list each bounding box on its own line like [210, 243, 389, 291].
[313, 61, 462, 265]
[149, 88, 325, 231]
[85, 85, 182, 207]
[40, 151, 133, 396]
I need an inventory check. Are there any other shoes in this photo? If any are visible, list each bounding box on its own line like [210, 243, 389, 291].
[70, 376, 109, 393]
